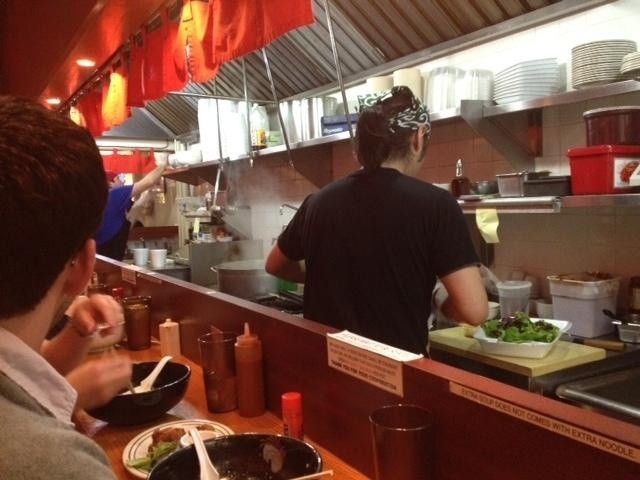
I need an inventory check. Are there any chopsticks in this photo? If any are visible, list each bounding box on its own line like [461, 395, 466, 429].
[109, 344, 136, 394]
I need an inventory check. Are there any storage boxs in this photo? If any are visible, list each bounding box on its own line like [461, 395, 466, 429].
[545, 269, 622, 339]
[566, 104, 640, 194]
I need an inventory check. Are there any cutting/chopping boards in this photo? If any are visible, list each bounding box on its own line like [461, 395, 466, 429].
[430, 326, 606, 377]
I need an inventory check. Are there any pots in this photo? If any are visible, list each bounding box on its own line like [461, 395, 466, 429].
[278, 97, 337, 145]
[210, 260, 281, 300]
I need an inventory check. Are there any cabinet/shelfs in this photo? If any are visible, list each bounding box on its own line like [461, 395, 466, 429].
[427, 76, 640, 209]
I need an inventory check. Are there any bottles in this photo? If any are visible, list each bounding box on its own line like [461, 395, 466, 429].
[112, 288, 125, 304]
[249, 102, 267, 150]
[234, 323, 265, 418]
[452, 159, 471, 197]
[367, 60, 493, 115]
[159, 319, 180, 359]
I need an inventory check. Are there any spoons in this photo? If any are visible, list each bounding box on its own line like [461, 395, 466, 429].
[134, 355, 173, 392]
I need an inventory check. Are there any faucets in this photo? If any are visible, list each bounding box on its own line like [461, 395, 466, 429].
[279, 203, 299, 216]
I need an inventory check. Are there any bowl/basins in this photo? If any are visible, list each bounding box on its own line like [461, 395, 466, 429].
[84, 361, 323, 480]
[471, 180, 499, 195]
[488, 298, 553, 320]
[87, 321, 125, 353]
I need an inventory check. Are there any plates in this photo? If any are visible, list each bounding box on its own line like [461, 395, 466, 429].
[169, 149, 202, 169]
[494, 40, 640, 105]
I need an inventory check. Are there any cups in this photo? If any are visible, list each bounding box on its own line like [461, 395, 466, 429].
[88, 284, 108, 297]
[198, 331, 238, 413]
[368, 404, 438, 479]
[124, 295, 151, 350]
[496, 280, 533, 319]
[133, 248, 167, 268]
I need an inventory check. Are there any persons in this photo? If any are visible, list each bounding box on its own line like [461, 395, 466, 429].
[266, 85, 491, 359]
[41, 293, 133, 421]
[96, 152, 169, 262]
[0, 97, 130, 480]
